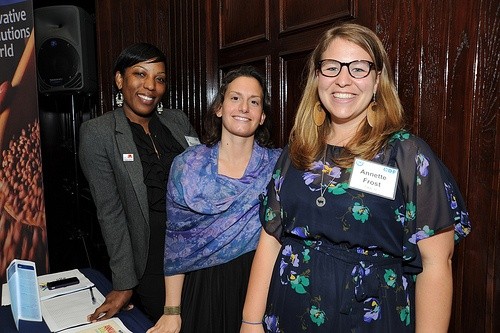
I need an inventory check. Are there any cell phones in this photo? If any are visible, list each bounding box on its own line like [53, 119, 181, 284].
[46, 277, 79, 290]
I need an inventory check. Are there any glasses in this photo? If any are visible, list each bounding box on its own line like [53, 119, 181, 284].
[316, 59, 375, 78]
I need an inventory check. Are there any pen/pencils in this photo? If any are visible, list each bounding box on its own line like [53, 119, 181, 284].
[89, 286, 95, 304]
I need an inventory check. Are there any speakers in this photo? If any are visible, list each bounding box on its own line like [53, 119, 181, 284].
[33, 5, 96, 97]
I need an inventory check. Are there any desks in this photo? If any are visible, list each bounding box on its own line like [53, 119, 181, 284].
[0, 268, 154, 333]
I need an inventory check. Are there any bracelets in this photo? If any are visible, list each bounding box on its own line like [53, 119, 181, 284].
[163, 305, 181, 315]
[241, 320, 262, 324]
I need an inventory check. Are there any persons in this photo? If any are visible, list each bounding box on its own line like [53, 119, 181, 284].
[77, 42, 205, 325]
[145, 65, 283, 333]
[237, 23, 471, 333]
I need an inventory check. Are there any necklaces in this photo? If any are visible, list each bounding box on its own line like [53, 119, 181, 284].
[315, 139, 351, 207]
